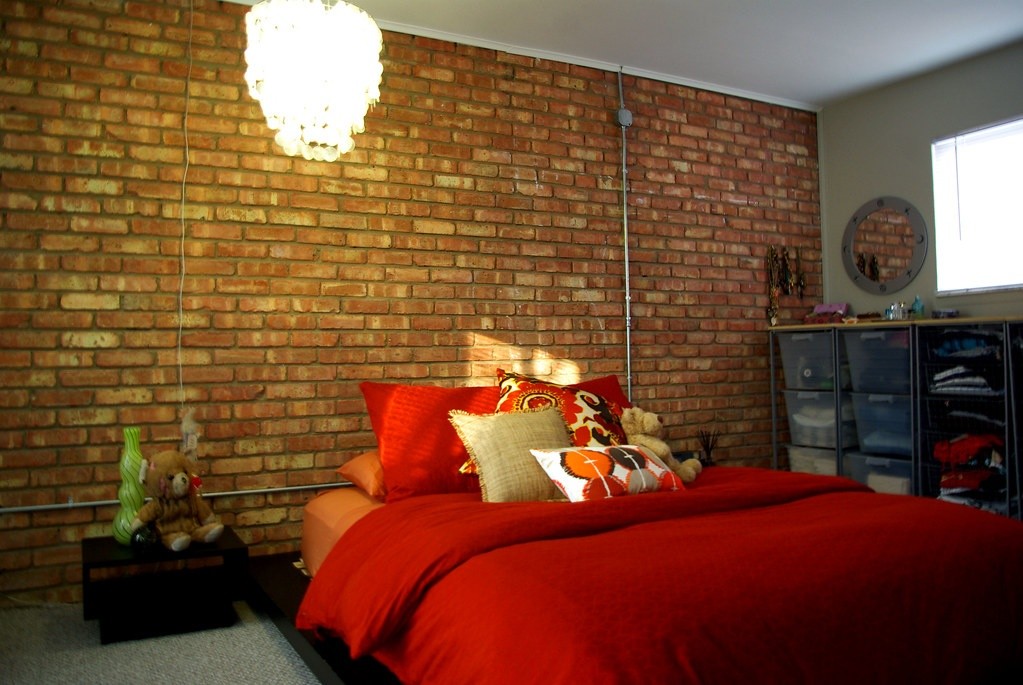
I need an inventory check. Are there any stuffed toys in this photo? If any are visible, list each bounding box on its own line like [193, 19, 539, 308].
[620, 406, 702, 481]
[130, 449, 224, 551]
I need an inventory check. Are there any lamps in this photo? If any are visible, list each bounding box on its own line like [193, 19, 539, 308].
[242, 0, 385, 164]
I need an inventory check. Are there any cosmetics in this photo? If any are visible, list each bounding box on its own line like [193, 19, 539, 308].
[884, 294, 924, 321]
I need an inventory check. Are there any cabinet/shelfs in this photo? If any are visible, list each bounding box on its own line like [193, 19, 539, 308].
[769, 317, 1023, 523]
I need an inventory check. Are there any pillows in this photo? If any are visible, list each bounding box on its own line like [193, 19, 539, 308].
[448, 407, 576, 502]
[530, 444, 687, 505]
[334, 449, 388, 505]
[357, 372, 632, 507]
[458, 367, 627, 474]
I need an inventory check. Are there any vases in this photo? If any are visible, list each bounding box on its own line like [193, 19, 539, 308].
[111, 428, 145, 547]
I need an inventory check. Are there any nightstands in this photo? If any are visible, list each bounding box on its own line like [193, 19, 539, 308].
[79, 525, 247, 645]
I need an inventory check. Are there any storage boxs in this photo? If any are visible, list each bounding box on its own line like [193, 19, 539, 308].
[780, 330, 911, 493]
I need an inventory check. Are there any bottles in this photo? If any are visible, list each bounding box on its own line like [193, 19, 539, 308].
[888, 300, 909, 322]
[912, 295, 925, 315]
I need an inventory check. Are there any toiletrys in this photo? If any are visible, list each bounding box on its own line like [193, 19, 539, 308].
[884, 293, 923, 320]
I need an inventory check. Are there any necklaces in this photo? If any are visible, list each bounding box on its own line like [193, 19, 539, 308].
[779, 248, 795, 296]
[794, 250, 809, 299]
[766, 247, 781, 326]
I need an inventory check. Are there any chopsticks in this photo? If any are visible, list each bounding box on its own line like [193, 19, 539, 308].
[695, 428, 722, 457]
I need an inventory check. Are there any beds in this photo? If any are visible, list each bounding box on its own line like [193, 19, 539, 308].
[295, 465, 1022, 684]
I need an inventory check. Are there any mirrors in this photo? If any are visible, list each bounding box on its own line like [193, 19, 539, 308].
[844, 195, 928, 294]
